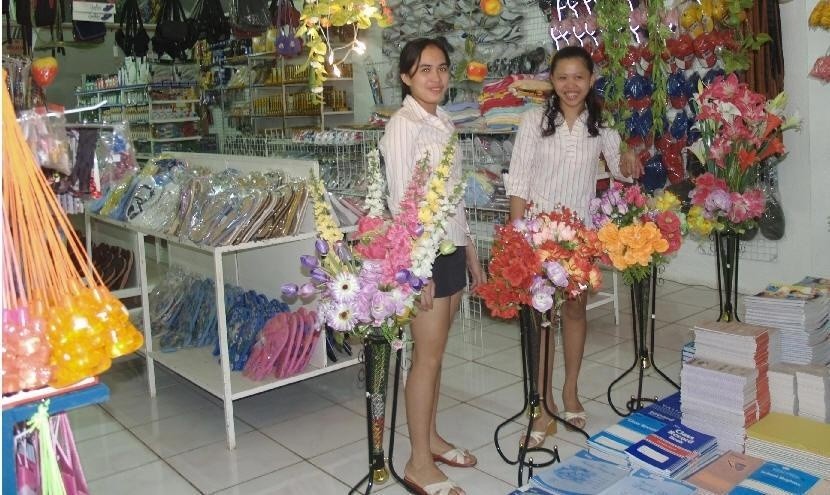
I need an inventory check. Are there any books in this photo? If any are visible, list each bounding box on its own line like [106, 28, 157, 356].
[507, 392, 679, 493]
[680, 277, 830, 495]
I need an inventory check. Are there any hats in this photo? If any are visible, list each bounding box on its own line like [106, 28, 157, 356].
[758, 197, 785, 241]
[738, 225, 757, 240]
[541, 0, 740, 212]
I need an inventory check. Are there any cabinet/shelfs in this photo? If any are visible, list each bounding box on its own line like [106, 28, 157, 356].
[219, 50, 357, 161]
[323, 127, 620, 329]
[2, 382, 112, 495]
[84, 151, 408, 451]
[76, 80, 201, 162]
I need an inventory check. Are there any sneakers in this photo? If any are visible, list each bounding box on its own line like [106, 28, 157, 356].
[381, 0, 524, 59]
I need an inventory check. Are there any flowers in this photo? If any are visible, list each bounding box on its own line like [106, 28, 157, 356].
[280, 129, 473, 354]
[475, 199, 613, 320]
[585, 180, 685, 286]
[686, 72, 806, 236]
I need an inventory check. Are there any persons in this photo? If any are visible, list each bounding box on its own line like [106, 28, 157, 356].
[376, 37, 490, 495]
[504, 46, 645, 451]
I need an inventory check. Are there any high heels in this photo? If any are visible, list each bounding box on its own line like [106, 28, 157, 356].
[518, 409, 559, 452]
[565, 409, 586, 432]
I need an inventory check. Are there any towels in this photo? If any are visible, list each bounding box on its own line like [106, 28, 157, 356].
[445, 73, 552, 129]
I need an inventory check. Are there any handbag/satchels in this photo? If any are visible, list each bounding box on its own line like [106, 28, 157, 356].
[13, 411, 88, 494]
[114, 0, 302, 58]
[72, 20, 107, 42]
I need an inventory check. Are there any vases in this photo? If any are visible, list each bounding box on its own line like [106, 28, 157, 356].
[493, 303, 591, 489]
[607, 262, 681, 419]
[713, 229, 742, 323]
[345, 324, 425, 495]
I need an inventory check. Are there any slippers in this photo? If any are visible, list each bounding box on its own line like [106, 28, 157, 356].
[433, 443, 477, 468]
[70, 230, 135, 292]
[141, 269, 321, 381]
[402, 475, 467, 495]
[91, 153, 309, 248]
